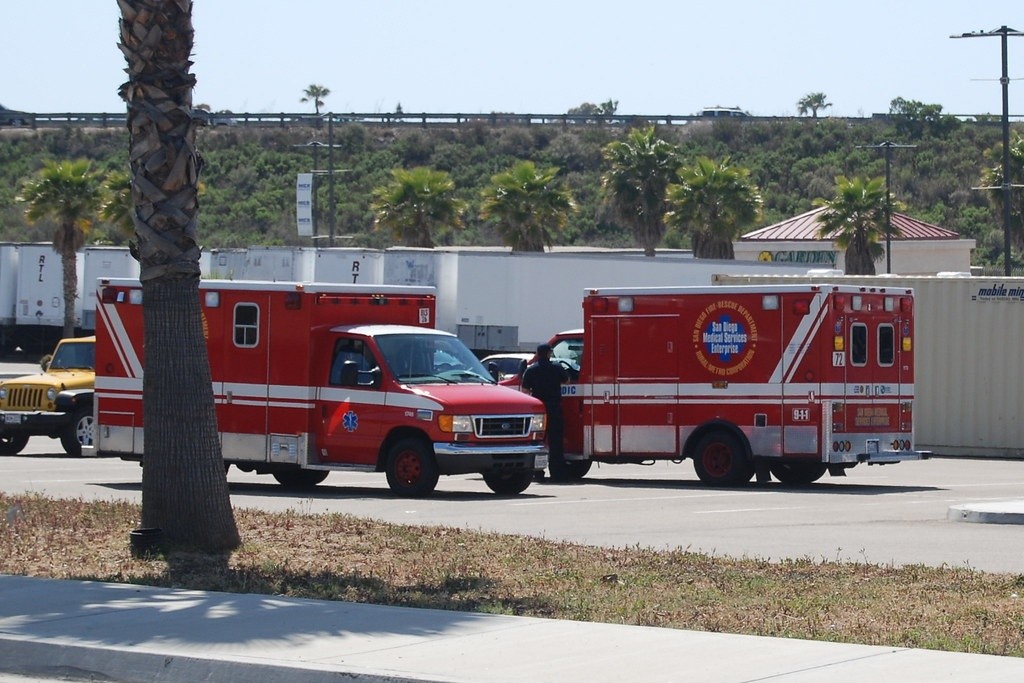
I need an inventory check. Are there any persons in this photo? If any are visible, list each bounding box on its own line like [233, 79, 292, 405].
[520, 344, 568, 483]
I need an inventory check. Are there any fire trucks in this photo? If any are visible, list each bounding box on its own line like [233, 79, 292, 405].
[499, 284, 916, 487]
[92, 277, 547, 498]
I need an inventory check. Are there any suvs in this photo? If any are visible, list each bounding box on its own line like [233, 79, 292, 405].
[0, 338, 96, 458]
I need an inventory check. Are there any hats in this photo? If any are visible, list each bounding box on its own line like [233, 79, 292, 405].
[537, 344, 553, 354]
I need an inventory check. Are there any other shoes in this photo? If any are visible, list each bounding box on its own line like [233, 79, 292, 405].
[550, 475, 566, 483]
[534, 474, 546, 482]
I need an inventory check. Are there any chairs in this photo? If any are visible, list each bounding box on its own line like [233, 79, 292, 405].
[331, 345, 373, 384]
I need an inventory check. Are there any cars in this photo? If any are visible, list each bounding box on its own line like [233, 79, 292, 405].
[466, 353, 536, 393]
